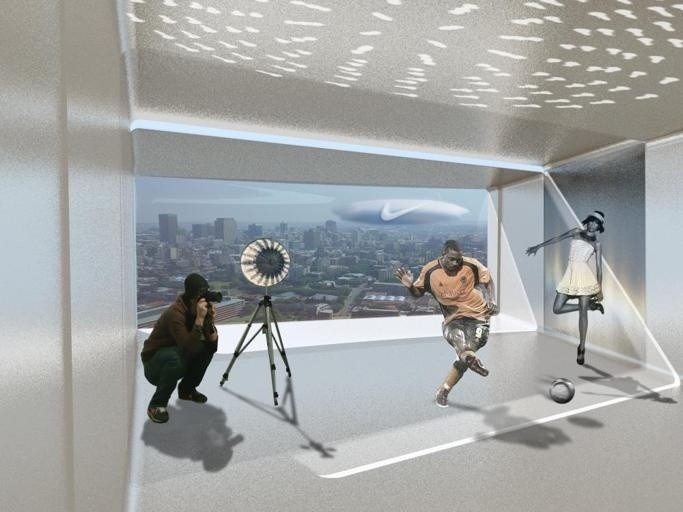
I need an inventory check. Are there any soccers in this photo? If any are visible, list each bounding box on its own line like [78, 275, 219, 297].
[550, 377, 573, 404]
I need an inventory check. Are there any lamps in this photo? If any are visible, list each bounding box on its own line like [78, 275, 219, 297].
[239, 239, 290, 288]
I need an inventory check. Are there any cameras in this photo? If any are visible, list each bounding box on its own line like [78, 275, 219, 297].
[197, 288, 223, 306]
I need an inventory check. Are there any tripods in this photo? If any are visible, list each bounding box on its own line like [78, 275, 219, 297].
[219, 293, 291, 407]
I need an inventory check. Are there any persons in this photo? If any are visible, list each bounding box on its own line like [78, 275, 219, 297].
[524, 209, 604, 366]
[394, 240, 500, 409]
[141, 272, 222, 422]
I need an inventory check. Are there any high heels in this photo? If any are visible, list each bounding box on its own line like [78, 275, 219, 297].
[576, 343, 585, 364]
[589, 299, 604, 314]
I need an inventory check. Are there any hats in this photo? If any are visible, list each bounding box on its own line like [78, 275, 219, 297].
[587, 211, 605, 224]
[183, 274, 209, 298]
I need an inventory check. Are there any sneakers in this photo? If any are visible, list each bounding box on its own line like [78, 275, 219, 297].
[147, 405, 169, 423]
[433, 387, 450, 409]
[178, 388, 207, 403]
[461, 351, 489, 377]
